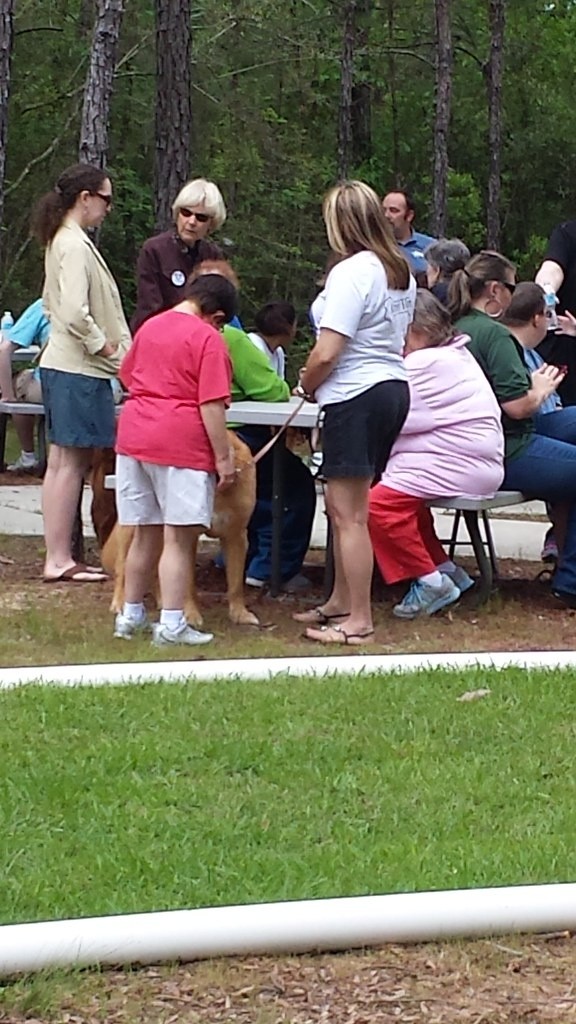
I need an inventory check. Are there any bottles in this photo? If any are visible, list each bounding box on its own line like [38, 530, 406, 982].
[0, 312, 14, 335]
[542, 281, 558, 330]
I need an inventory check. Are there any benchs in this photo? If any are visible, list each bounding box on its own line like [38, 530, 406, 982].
[423, 490, 535, 610]
[0, 401, 123, 479]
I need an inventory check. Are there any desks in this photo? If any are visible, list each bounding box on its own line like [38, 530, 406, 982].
[12, 345, 41, 361]
[224, 393, 336, 599]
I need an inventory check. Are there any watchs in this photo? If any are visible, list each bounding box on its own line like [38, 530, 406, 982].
[297, 380, 311, 399]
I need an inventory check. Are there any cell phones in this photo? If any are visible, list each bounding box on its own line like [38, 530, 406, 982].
[553, 365, 569, 381]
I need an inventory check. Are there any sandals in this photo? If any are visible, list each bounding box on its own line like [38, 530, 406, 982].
[301, 626, 375, 647]
[292, 607, 351, 625]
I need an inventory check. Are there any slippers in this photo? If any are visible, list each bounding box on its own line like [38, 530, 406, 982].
[44, 561, 108, 583]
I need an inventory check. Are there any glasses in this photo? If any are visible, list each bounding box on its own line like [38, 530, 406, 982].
[544, 311, 553, 319]
[502, 281, 517, 295]
[180, 207, 211, 223]
[88, 191, 112, 205]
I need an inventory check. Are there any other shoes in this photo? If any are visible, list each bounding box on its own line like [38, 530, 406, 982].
[245, 573, 310, 592]
[541, 541, 558, 561]
[6, 451, 37, 471]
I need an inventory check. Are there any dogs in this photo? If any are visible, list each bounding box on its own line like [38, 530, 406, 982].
[90, 426, 261, 629]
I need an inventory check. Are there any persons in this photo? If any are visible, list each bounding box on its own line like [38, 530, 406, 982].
[183, 258, 317, 593]
[114, 274, 237, 645]
[292, 180, 417, 644]
[367, 191, 576, 619]
[29, 164, 134, 582]
[0, 297, 54, 471]
[91, 179, 227, 549]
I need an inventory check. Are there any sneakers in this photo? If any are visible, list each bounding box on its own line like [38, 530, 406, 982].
[151, 616, 215, 648]
[112, 612, 158, 641]
[393, 566, 475, 618]
